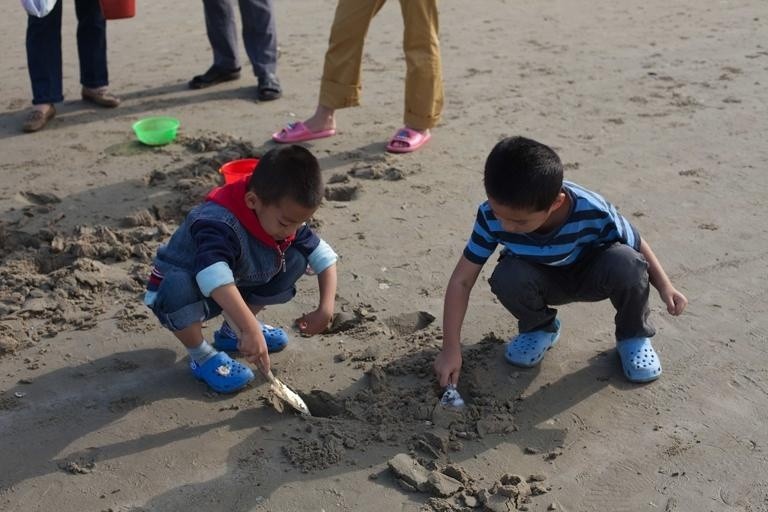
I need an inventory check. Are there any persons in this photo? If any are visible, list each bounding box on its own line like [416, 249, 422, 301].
[21, 0, 120, 134]
[143, 144, 339, 394]
[189, 0, 282, 101]
[273, 0, 444, 154]
[434, 135, 687, 390]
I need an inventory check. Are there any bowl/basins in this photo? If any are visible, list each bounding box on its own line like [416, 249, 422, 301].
[220, 159, 259, 184]
[131, 115, 179, 146]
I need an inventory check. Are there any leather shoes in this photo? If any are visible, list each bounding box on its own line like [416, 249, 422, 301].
[21, 104, 58, 133]
[79, 85, 121, 109]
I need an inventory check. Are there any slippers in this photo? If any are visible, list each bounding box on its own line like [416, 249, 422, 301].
[503, 317, 562, 368]
[271, 121, 337, 143]
[385, 127, 433, 156]
[211, 322, 289, 354]
[257, 75, 283, 103]
[188, 66, 243, 90]
[188, 348, 256, 396]
[614, 337, 663, 383]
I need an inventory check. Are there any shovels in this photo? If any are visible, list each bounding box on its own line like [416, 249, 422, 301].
[439, 382, 465, 409]
[220, 309, 311, 416]
